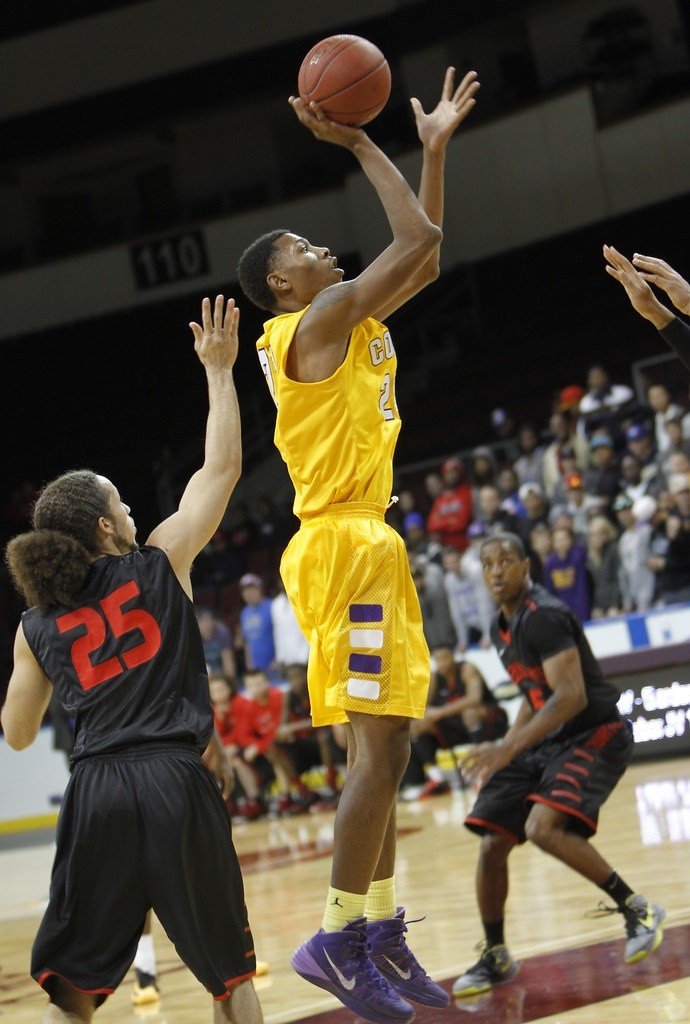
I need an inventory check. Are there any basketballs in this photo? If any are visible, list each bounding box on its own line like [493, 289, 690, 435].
[297, 31, 392, 129]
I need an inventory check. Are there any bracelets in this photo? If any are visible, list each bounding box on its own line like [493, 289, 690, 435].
[503, 733, 529, 752]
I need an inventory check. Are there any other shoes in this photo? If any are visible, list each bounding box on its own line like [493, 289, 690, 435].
[416, 779, 450, 801]
[220, 786, 339, 824]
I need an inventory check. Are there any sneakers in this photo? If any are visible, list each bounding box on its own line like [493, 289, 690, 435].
[453, 940, 519, 997]
[129, 978, 160, 1004]
[583, 892, 667, 962]
[290, 915, 415, 1024]
[355, 906, 449, 1007]
[253, 960, 271, 978]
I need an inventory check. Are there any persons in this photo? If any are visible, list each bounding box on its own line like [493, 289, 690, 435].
[603, 245, 690, 370]
[193, 370, 690, 824]
[0, 294, 275, 1023]
[453, 534, 668, 997]
[131, 907, 269, 1004]
[235, 67, 480, 1024]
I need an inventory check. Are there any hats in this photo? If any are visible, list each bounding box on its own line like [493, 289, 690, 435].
[519, 474, 583, 498]
[552, 423, 651, 453]
[612, 496, 633, 511]
[557, 386, 582, 412]
[430, 443, 512, 470]
[241, 574, 263, 587]
